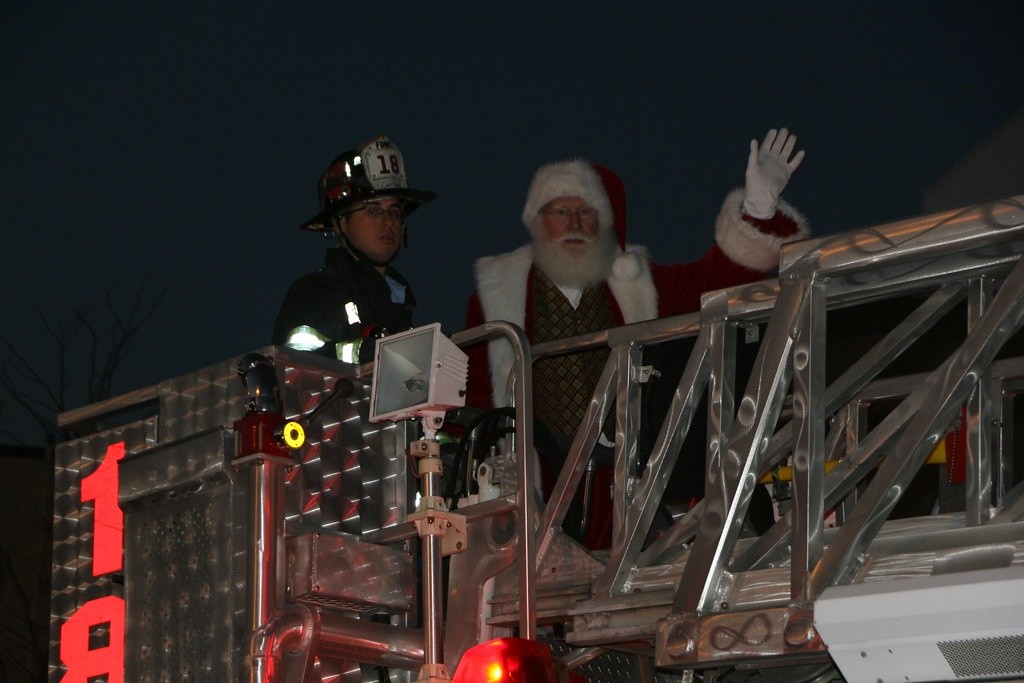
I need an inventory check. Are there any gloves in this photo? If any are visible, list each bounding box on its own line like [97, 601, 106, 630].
[743, 127, 805, 220]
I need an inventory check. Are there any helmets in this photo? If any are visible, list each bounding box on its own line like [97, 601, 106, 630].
[297, 135, 436, 234]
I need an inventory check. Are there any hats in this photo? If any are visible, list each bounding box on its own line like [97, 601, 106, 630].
[520, 158, 644, 281]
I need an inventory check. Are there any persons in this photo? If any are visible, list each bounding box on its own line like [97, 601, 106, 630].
[274, 135, 439, 366]
[444, 127, 812, 547]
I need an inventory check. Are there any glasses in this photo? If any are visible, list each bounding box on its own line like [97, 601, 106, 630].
[548, 205, 597, 220]
[338, 205, 403, 221]
